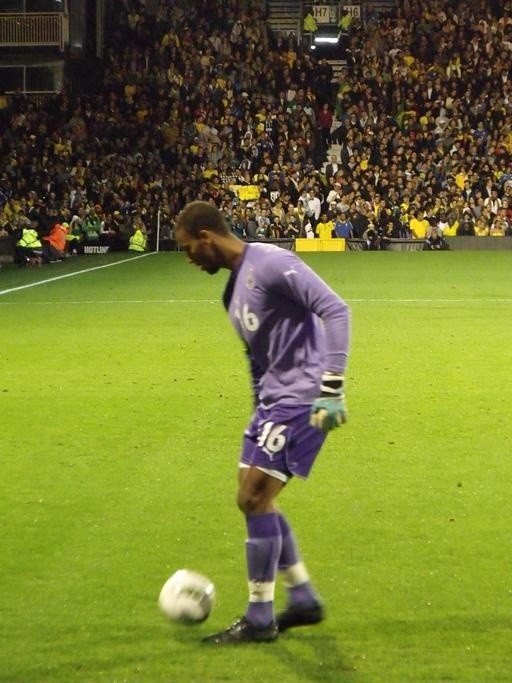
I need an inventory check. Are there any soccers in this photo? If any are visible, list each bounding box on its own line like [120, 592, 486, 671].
[157, 569, 216, 628]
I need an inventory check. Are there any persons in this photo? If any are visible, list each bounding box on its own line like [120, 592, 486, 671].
[176, 200, 349, 647]
[1, 0, 511, 266]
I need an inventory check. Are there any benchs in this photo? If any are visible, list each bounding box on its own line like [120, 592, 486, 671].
[264, 0, 398, 82]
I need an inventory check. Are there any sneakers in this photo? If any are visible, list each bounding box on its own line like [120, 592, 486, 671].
[276, 603, 323, 631]
[204, 616, 278, 645]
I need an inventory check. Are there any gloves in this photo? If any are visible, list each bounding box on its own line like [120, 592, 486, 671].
[308, 374, 347, 432]
